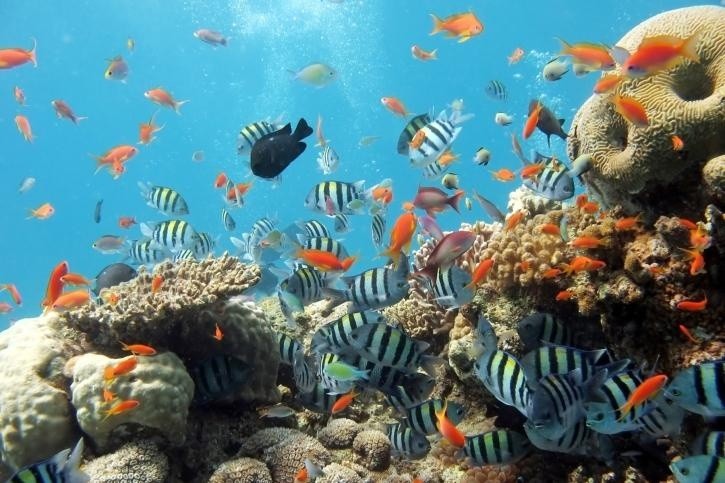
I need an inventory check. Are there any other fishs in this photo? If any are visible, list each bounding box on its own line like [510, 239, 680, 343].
[0, 28, 725, 483]
